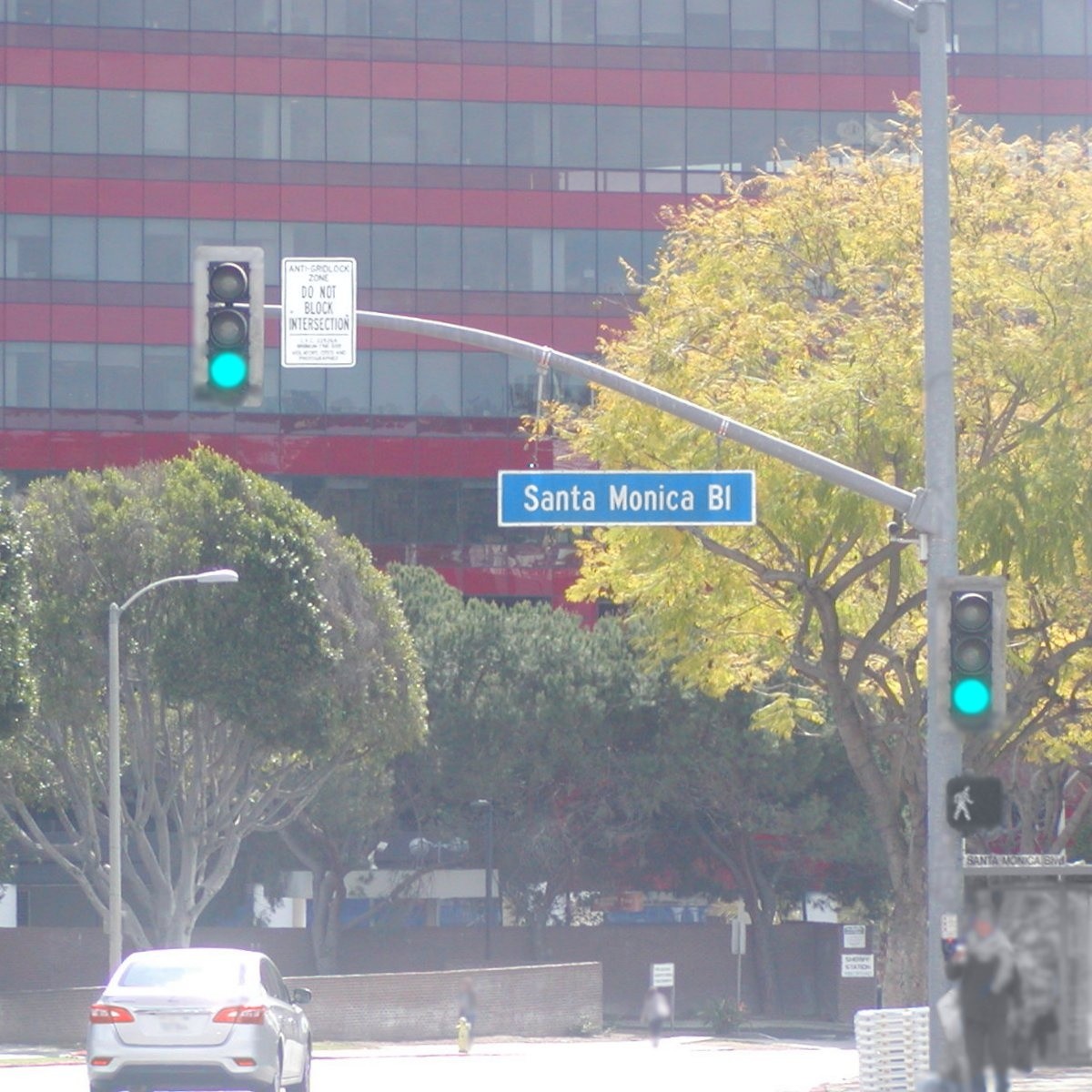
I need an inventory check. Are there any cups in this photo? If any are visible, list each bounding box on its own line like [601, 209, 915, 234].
[955, 946, 966, 959]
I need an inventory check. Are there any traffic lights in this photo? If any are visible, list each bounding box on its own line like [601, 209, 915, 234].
[946, 772, 1003, 834]
[946, 574, 1007, 727]
[188, 246, 264, 404]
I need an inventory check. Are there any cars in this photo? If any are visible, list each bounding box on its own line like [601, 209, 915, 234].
[86, 946, 313, 1092]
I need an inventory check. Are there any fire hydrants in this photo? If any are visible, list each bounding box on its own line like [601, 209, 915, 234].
[456, 1016, 472, 1054]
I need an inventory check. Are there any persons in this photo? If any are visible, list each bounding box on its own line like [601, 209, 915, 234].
[944, 908, 1023, 1092]
[640, 987, 671, 1048]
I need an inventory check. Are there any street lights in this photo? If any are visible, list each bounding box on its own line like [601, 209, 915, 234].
[107, 567, 239, 972]
[466, 799, 495, 968]
